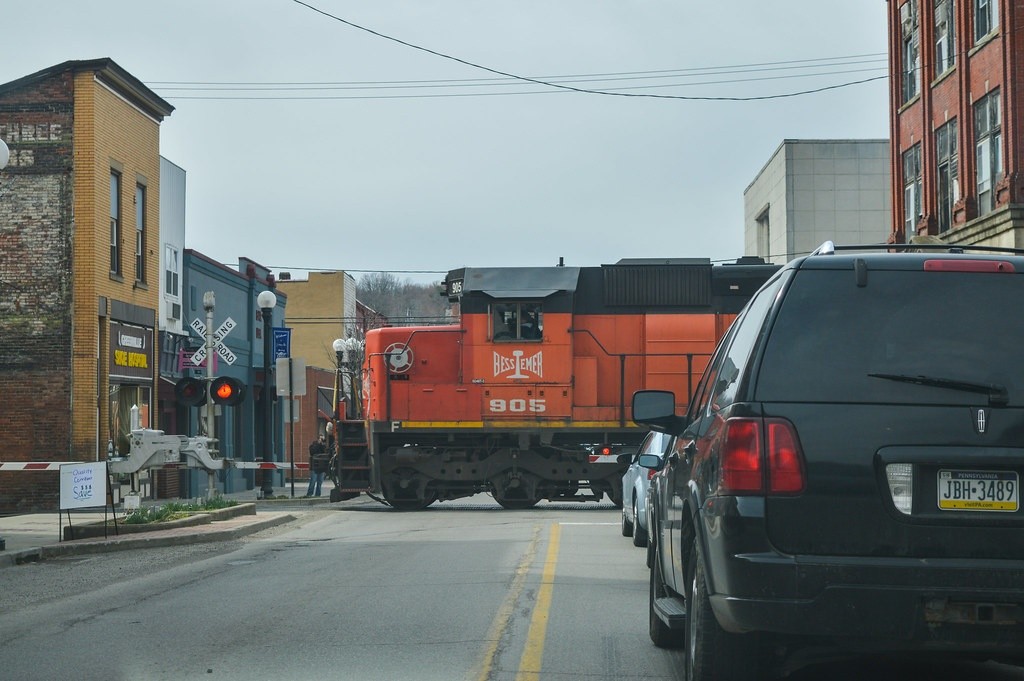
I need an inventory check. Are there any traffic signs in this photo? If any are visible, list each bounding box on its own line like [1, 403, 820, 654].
[189, 317, 238, 367]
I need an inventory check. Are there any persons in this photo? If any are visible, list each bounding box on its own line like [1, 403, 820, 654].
[303, 433, 328, 499]
[510, 308, 543, 340]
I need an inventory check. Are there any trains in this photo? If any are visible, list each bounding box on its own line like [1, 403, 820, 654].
[330, 255, 786, 513]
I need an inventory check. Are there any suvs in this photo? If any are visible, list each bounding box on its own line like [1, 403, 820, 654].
[628, 238, 1023, 681]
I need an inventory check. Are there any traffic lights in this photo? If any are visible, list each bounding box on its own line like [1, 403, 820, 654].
[175, 375, 247, 409]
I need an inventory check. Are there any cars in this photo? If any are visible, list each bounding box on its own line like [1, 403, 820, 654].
[616, 429, 672, 548]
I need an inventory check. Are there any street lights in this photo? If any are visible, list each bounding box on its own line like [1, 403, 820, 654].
[334, 338, 366, 422]
[256, 290, 278, 499]
